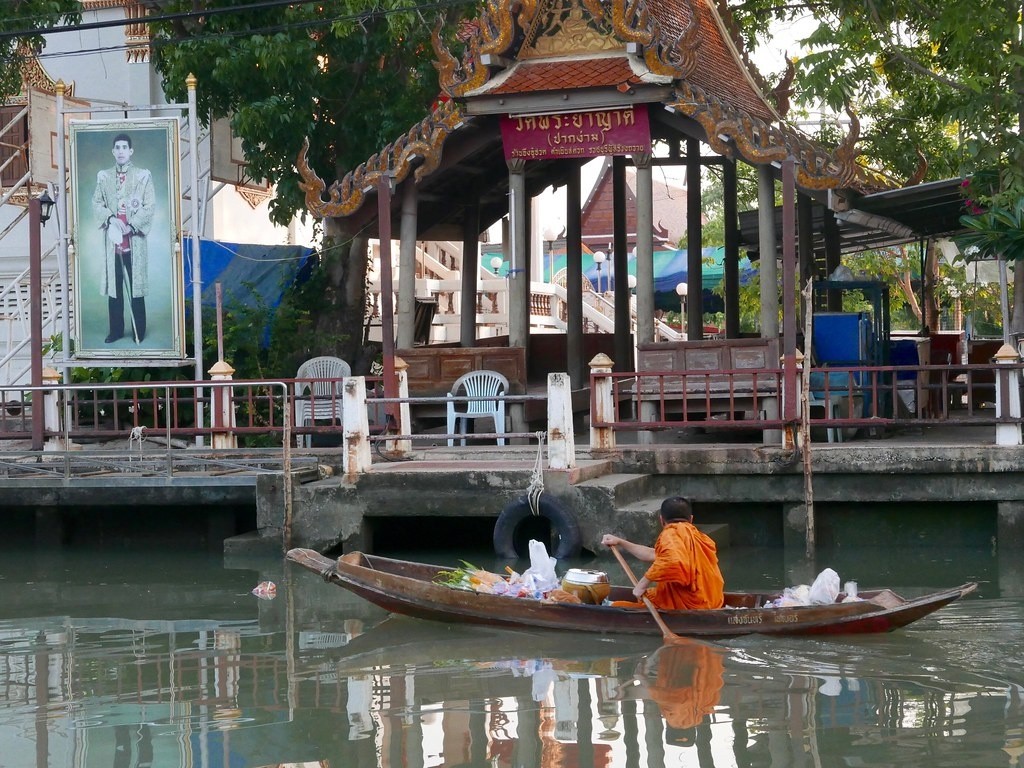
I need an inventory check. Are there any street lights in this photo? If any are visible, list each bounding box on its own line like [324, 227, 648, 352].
[675, 281, 687, 333]
[628, 276, 636, 332]
[593, 252, 606, 293]
[542, 225, 558, 284]
[29, 189, 54, 451]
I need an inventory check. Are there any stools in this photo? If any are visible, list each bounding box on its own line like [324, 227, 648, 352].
[809, 398, 841, 443]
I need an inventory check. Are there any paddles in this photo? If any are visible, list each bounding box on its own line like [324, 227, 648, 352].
[607, 544, 680, 642]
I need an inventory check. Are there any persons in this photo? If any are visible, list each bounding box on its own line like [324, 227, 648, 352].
[601, 497, 724, 612]
[92, 134, 156, 344]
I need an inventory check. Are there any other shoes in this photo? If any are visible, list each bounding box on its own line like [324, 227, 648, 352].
[134, 335, 144, 343]
[105, 333, 120, 343]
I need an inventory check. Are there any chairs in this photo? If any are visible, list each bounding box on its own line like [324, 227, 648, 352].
[445, 370, 510, 447]
[296, 356, 351, 447]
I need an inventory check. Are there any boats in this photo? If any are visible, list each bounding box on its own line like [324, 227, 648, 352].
[286, 547, 979, 640]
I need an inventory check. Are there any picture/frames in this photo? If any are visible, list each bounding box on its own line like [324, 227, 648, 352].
[67, 118, 187, 359]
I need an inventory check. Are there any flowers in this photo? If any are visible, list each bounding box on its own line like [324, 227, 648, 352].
[433, 559, 509, 596]
